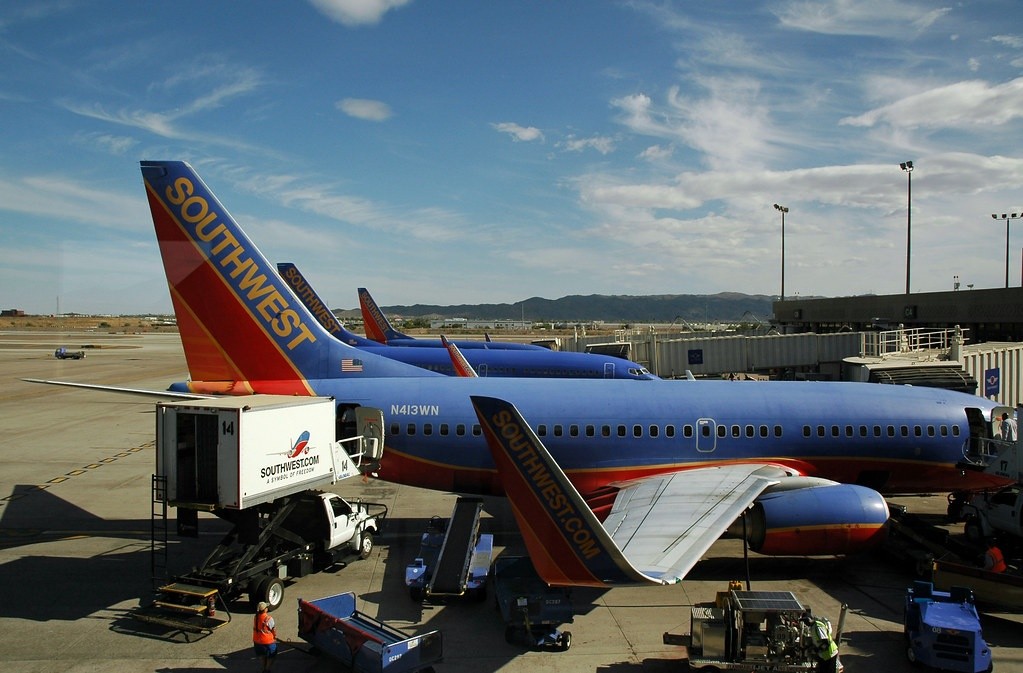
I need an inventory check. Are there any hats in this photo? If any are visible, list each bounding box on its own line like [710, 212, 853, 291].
[257, 602, 270, 611]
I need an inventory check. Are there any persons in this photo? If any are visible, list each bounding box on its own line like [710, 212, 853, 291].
[253, 602, 277, 672]
[977, 538, 1008, 574]
[729, 372, 734, 381]
[743, 374, 755, 380]
[799, 612, 839, 673]
[1001, 413, 1017, 441]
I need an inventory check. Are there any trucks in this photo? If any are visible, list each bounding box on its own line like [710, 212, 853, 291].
[124, 488, 391, 644]
[55, 348, 86, 360]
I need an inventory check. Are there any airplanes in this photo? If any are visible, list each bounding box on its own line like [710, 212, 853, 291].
[18, 162, 1023, 588]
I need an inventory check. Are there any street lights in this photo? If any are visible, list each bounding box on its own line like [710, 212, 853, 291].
[899, 163, 914, 295]
[990, 213, 1023, 288]
[773, 206, 789, 300]
